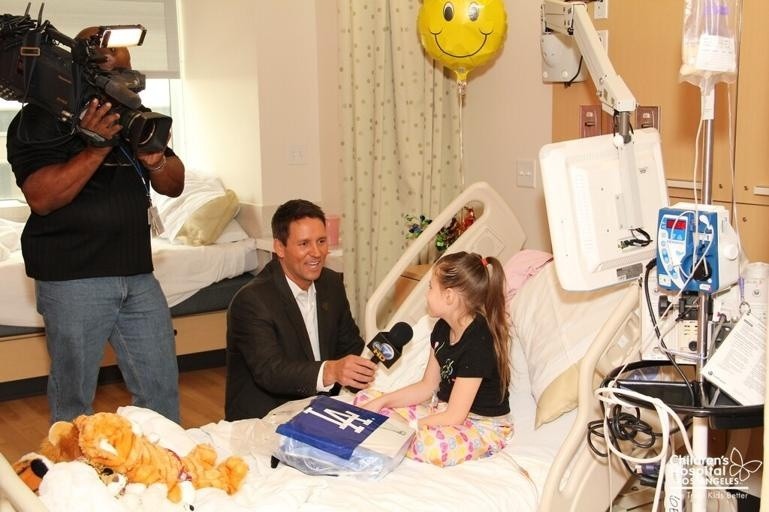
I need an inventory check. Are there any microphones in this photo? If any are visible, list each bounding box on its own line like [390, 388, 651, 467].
[347, 322, 413, 393]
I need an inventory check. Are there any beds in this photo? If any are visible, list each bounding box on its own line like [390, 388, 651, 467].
[1, 182, 695, 512]
[0, 212, 257, 403]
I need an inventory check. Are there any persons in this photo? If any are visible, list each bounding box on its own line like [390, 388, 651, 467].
[360, 250, 513, 467]
[5, 27, 188, 425]
[223, 198, 378, 424]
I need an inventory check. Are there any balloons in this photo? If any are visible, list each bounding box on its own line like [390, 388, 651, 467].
[416, 0, 508, 92]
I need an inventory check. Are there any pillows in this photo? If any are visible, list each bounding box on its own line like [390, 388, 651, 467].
[505, 258, 642, 430]
[147, 169, 250, 246]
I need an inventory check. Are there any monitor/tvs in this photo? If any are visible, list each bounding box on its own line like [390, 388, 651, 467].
[639, 261, 769, 362]
[538, 127, 670, 291]
